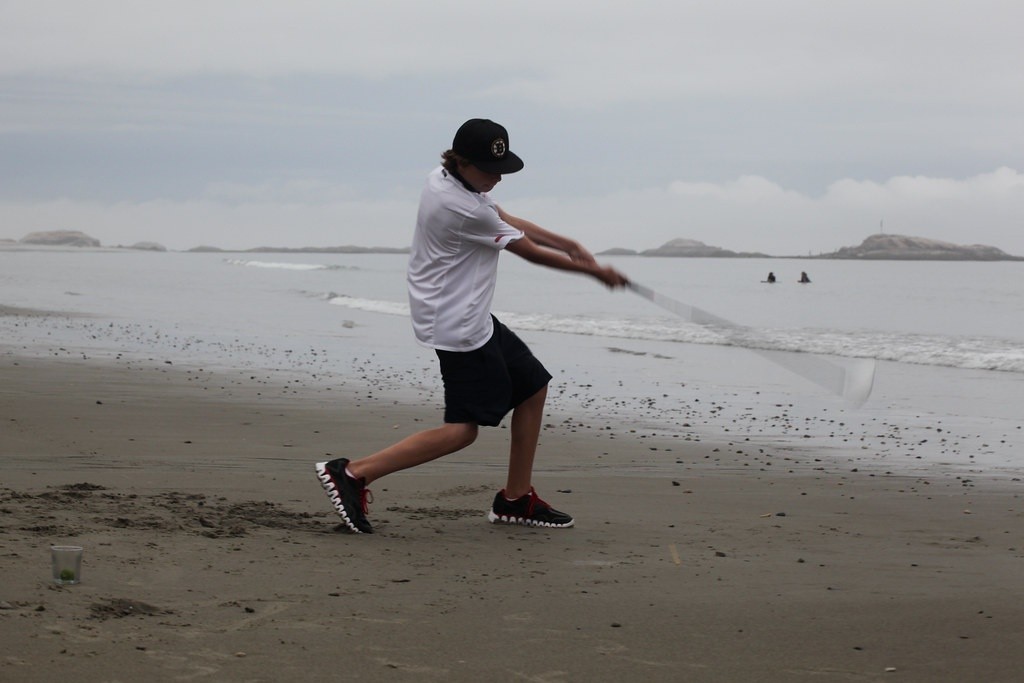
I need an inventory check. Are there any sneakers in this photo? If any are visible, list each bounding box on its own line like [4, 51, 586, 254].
[315, 458, 373, 534]
[488, 486, 576, 527]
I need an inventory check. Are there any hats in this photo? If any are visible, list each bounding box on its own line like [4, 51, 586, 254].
[453, 118, 524, 174]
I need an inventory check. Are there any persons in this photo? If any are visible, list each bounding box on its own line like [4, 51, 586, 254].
[314, 117, 628, 535]
[767, 273, 776, 282]
[801, 272, 810, 282]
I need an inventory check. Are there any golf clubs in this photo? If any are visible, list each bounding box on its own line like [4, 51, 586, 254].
[625, 280, 875, 404]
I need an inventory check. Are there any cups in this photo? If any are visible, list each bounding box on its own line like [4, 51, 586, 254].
[51, 546, 83, 584]
